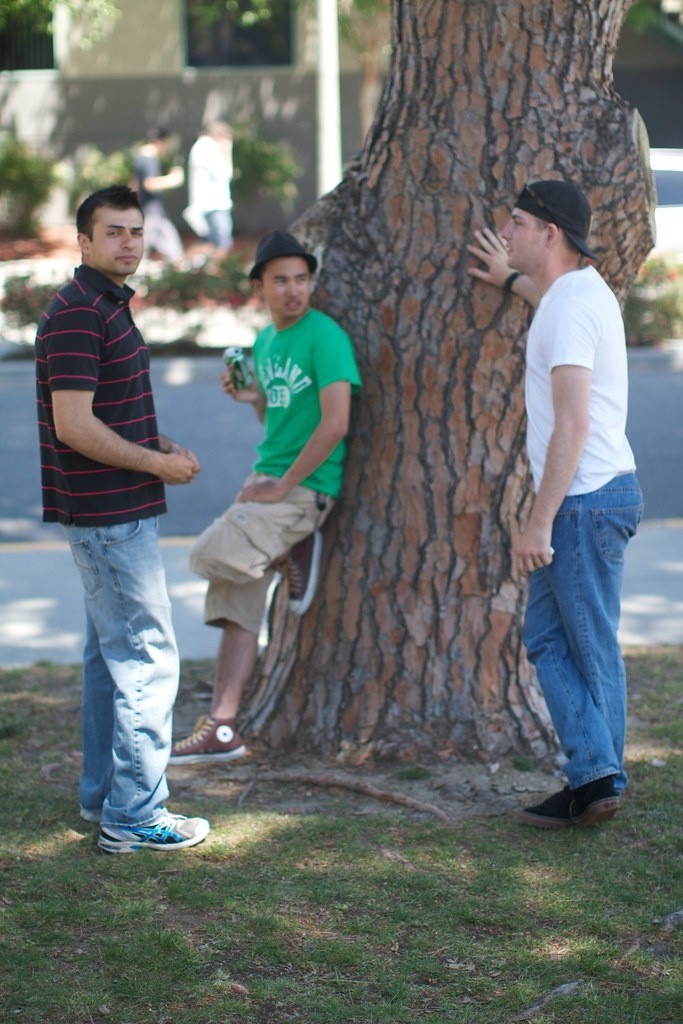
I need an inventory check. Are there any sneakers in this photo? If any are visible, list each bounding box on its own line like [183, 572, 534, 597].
[279, 531, 323, 616]
[98, 812, 209, 854]
[165, 713, 247, 765]
[79, 804, 102, 823]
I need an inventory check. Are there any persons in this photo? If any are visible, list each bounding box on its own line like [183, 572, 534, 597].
[33, 184, 211, 855]
[136, 120, 235, 262]
[465, 179, 644, 830]
[169, 229, 364, 766]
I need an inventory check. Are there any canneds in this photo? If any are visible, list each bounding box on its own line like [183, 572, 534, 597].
[222, 346, 252, 390]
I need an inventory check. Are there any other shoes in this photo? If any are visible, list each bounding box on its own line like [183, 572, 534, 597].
[570, 775, 619, 825]
[518, 786, 574, 829]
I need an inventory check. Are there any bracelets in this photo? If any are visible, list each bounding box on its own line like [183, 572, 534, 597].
[503, 271, 523, 295]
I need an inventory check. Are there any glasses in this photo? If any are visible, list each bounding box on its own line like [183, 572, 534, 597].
[518, 183, 560, 228]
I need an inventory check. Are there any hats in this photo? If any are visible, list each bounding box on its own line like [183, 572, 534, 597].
[514, 180, 595, 259]
[250, 230, 317, 280]
